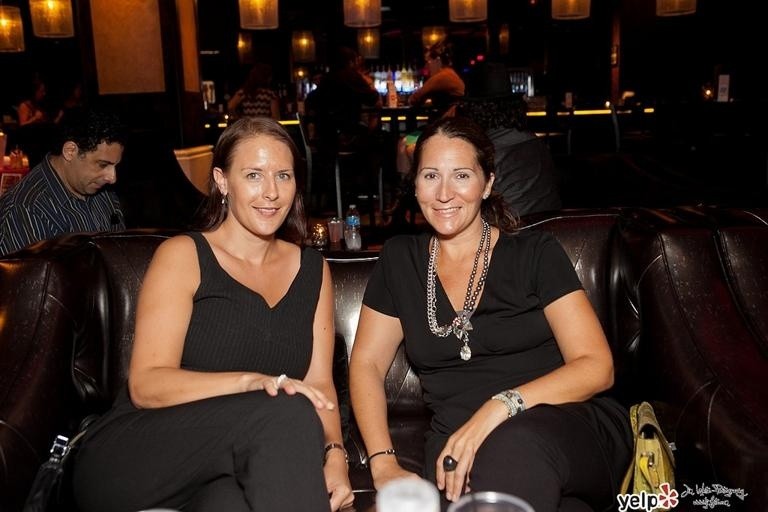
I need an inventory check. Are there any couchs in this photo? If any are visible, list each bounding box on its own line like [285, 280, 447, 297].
[1, 204, 768, 512]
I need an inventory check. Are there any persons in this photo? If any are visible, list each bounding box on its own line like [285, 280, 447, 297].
[63, 114, 357, 512]
[1, 110, 126, 259]
[53, 85, 80, 124]
[453, 77, 562, 223]
[346, 115, 635, 512]
[224, 43, 465, 223]
[13, 76, 53, 169]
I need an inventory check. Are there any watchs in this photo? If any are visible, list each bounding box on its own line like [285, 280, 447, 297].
[324, 442, 349, 464]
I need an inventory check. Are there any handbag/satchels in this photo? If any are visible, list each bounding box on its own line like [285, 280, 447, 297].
[621, 401, 677, 511]
[25, 415, 94, 512]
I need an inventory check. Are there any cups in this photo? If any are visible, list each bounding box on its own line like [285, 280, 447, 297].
[376, 477, 440, 511]
[312, 223, 327, 246]
[328, 221, 342, 242]
[446, 490, 534, 511]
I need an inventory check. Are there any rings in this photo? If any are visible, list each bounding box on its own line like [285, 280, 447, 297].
[276, 373, 287, 388]
[443, 455, 457, 471]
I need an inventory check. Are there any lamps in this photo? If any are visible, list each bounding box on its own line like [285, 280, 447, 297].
[233, 0, 702, 65]
[0, 0, 26, 53]
[29, 0, 74, 38]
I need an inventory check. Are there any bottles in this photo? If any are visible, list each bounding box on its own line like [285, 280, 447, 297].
[344, 203, 362, 250]
[362, 66, 425, 93]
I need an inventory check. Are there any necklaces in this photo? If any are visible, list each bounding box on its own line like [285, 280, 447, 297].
[426, 216, 491, 361]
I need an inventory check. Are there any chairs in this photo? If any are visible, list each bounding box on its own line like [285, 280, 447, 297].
[291, 106, 390, 224]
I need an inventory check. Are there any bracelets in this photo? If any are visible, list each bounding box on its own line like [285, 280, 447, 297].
[491, 389, 526, 417]
[367, 448, 400, 462]
